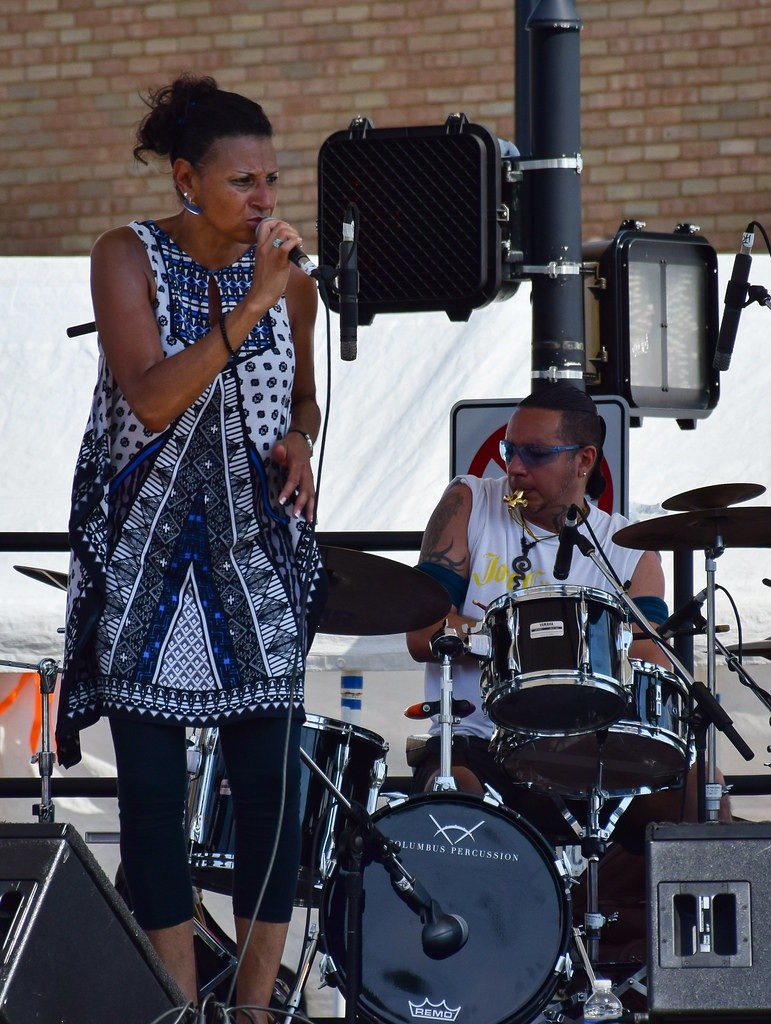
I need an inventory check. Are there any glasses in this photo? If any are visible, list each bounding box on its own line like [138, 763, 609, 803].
[497, 438, 586, 468]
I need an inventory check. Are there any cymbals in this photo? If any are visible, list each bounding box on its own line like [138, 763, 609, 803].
[609, 506, 771, 549]
[310, 543, 453, 638]
[700, 636, 771, 658]
[659, 482, 768, 511]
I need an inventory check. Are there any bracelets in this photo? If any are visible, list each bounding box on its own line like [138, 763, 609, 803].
[219, 316, 239, 358]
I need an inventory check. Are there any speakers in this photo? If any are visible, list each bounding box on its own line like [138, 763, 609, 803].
[645, 821, 771, 1024]
[0, 822, 197, 1024]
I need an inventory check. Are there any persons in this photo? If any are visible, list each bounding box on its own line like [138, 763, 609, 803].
[408, 383, 732, 820]
[57, 77, 322, 1024]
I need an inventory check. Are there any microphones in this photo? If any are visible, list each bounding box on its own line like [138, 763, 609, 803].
[552, 505, 578, 581]
[387, 865, 469, 957]
[256, 218, 320, 281]
[713, 223, 755, 371]
[657, 584, 719, 641]
[338, 208, 357, 363]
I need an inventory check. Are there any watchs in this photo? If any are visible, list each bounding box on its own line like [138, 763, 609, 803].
[290, 429, 312, 454]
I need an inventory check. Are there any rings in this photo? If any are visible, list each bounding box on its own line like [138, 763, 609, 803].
[273, 239, 283, 248]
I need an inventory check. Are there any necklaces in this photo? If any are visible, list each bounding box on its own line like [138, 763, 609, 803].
[502, 489, 587, 540]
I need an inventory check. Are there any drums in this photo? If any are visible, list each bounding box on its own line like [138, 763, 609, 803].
[317, 789, 573, 1024]
[182, 712, 391, 909]
[481, 583, 636, 737]
[497, 660, 693, 800]
